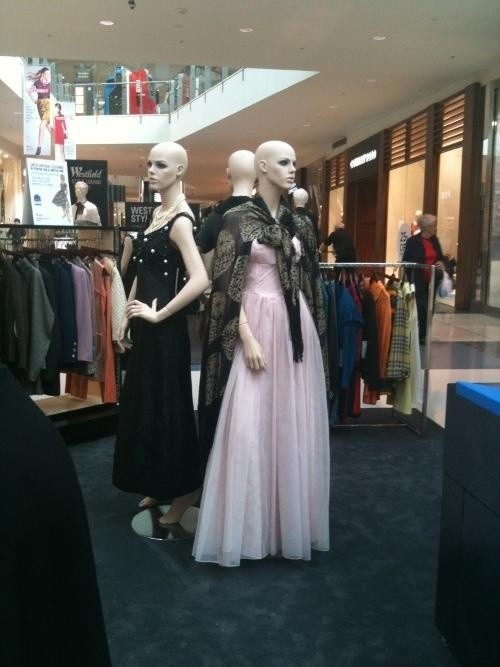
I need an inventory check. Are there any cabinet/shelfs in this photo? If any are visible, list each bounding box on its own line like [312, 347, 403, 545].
[435, 381, 499, 667]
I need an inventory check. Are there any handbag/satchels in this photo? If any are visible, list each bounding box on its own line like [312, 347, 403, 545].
[439, 271, 452, 297]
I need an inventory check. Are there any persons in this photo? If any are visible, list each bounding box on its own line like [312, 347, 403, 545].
[6, 219, 26, 251]
[112, 142, 210, 524]
[292, 188, 316, 233]
[52, 175, 70, 222]
[120, 235, 135, 299]
[72, 181, 102, 226]
[411, 210, 423, 237]
[24, 68, 68, 159]
[192, 140, 331, 569]
[319, 223, 358, 283]
[403, 214, 449, 348]
[196, 150, 257, 305]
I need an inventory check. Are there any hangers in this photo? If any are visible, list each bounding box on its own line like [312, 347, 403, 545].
[317, 261, 418, 283]
[0, 223, 101, 255]
